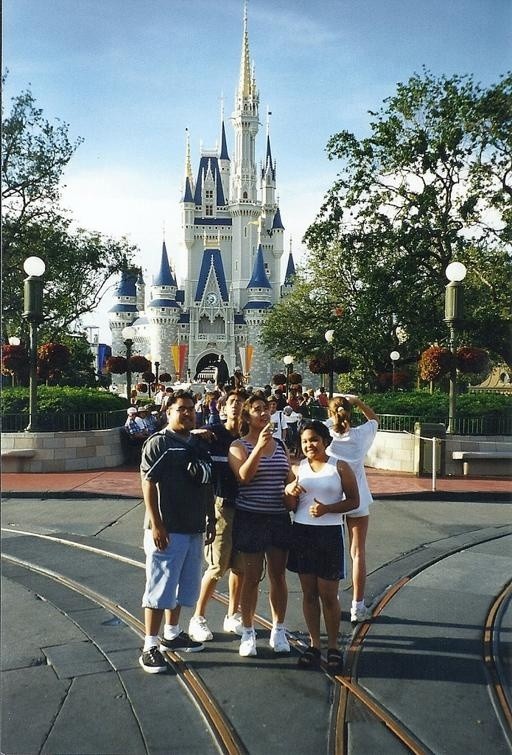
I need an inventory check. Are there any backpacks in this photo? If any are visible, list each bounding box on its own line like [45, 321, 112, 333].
[140, 431, 216, 506]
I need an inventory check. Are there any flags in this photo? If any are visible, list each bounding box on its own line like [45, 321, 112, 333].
[170, 343, 188, 379]
[239, 345, 255, 379]
[98, 343, 107, 370]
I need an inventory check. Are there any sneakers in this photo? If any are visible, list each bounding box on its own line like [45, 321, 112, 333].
[160, 630, 205, 654]
[139, 645, 168, 674]
[188, 615, 214, 643]
[269, 626, 291, 653]
[223, 612, 258, 638]
[238, 629, 258, 657]
[350, 606, 372, 623]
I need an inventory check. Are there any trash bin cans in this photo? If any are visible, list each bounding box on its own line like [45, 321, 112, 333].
[413, 421, 446, 479]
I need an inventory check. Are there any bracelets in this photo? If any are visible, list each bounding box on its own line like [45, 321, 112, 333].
[207, 518, 218, 524]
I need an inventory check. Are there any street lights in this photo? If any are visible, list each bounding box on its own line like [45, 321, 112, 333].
[437, 258, 468, 435]
[387, 347, 400, 393]
[118, 325, 136, 406]
[323, 328, 339, 405]
[282, 352, 294, 402]
[20, 253, 50, 435]
[144, 351, 163, 404]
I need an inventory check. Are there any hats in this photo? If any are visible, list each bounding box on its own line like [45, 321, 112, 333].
[126, 403, 152, 416]
[267, 395, 278, 402]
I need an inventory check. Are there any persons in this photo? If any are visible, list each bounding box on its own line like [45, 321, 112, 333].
[226, 391, 298, 659]
[133, 387, 219, 677]
[187, 387, 246, 643]
[284, 416, 360, 675]
[323, 392, 381, 626]
[123, 379, 331, 461]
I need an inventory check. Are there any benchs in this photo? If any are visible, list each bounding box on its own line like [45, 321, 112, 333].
[452, 451, 511, 475]
[1, 448, 37, 473]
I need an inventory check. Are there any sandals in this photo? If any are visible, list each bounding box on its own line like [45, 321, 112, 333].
[327, 648, 343, 673]
[297, 646, 322, 668]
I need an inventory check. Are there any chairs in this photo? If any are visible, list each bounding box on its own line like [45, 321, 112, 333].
[120, 429, 145, 461]
